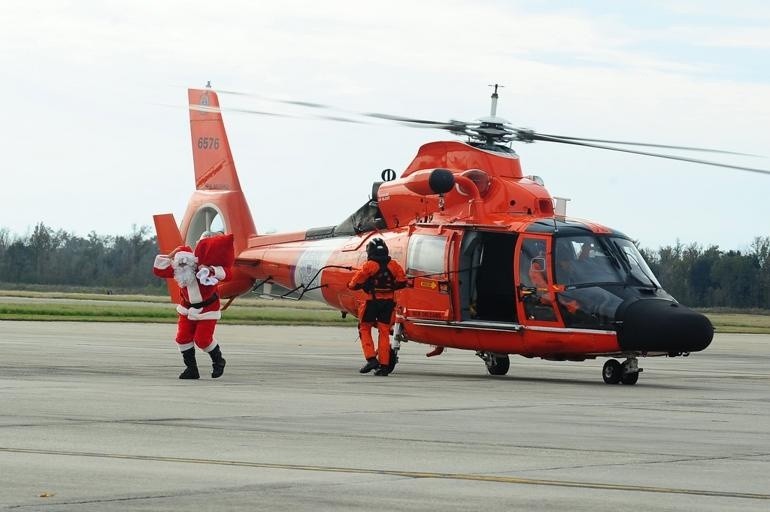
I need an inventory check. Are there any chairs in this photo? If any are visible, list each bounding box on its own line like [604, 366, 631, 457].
[359, 360, 389, 375]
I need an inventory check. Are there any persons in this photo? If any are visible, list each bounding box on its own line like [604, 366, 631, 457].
[529, 240, 583, 317]
[153, 244, 234, 379]
[347, 236, 408, 376]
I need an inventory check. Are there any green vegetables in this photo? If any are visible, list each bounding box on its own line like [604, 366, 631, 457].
[147, 84, 770, 387]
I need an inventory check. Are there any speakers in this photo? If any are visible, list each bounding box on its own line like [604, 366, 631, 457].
[179, 346, 200, 379]
[207, 345, 225, 378]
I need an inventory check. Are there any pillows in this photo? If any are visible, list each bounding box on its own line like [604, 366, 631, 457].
[366, 238, 388, 259]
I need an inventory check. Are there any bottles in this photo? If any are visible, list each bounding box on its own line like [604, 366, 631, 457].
[174, 246, 195, 260]
